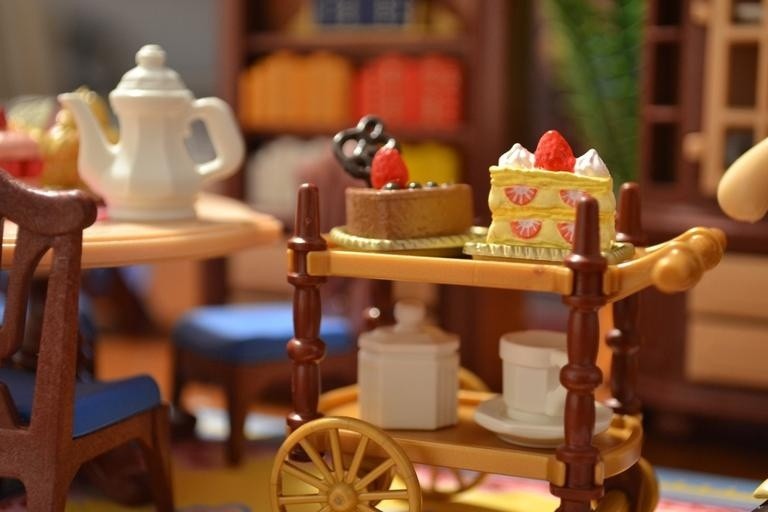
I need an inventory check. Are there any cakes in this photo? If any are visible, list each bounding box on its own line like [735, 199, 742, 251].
[327, 149, 487, 255]
[461, 128, 636, 265]
